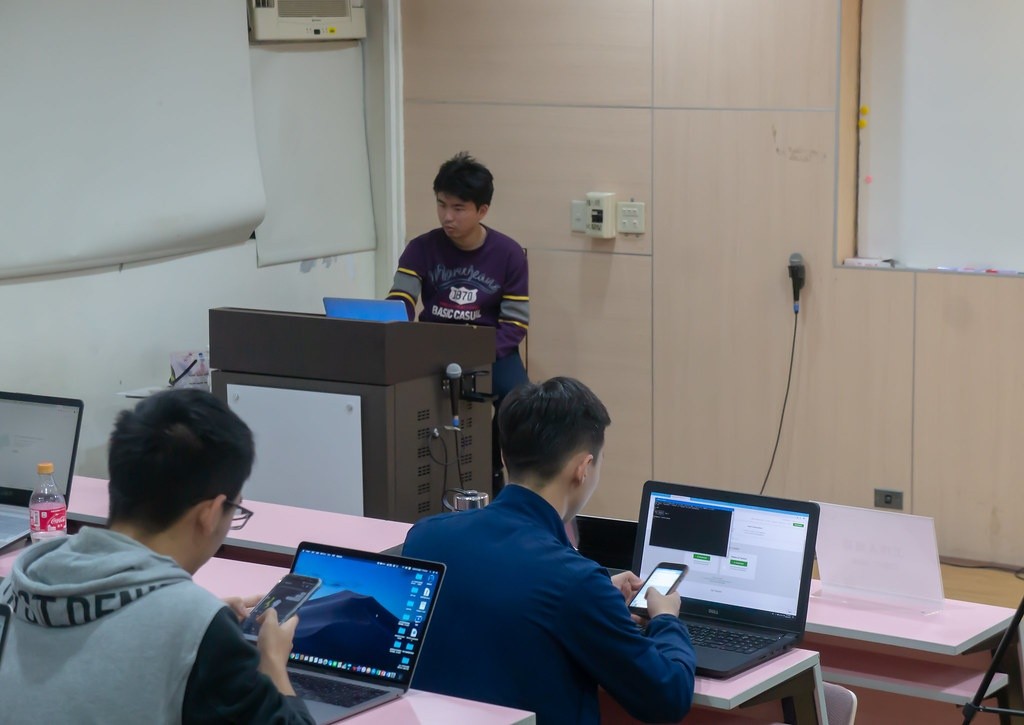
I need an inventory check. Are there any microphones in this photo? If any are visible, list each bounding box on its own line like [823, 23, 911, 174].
[447, 363, 462, 426]
[789, 253, 802, 314]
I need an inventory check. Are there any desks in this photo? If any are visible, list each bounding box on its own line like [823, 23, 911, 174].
[330, 686, 537, 725]
[0, 535, 828, 725]
[804, 577, 1024, 725]
[66, 474, 414, 556]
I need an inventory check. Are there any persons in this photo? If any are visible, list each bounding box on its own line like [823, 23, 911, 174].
[2, 384, 320, 724]
[394, 376, 698, 725]
[384, 157, 536, 503]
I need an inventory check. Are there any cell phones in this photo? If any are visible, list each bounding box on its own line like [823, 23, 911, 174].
[628, 562, 689, 614]
[240, 574, 322, 643]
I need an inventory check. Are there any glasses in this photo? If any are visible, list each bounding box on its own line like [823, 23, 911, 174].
[226, 499, 253, 530]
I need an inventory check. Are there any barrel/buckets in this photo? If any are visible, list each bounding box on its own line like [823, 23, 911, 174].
[167, 350, 208, 391]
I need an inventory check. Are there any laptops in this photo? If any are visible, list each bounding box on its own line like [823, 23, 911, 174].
[287, 542, 448, 725]
[633, 480, 820, 681]
[0, 391, 84, 550]
[323, 297, 409, 322]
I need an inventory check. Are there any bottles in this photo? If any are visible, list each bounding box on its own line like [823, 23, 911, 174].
[441, 488, 489, 512]
[28, 462, 67, 544]
